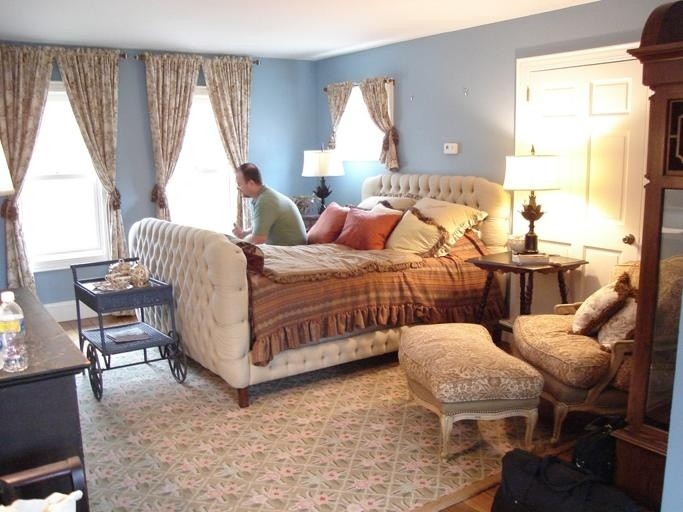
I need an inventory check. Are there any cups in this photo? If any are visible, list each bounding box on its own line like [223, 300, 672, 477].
[105, 272, 131, 288]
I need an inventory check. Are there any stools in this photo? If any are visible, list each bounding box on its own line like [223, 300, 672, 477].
[395, 318, 550, 464]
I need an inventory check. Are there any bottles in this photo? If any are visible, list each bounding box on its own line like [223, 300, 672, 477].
[0, 290, 29, 374]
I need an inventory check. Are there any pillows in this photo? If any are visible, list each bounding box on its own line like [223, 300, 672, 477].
[306, 196, 489, 258]
[596, 286, 641, 345]
[569, 278, 630, 337]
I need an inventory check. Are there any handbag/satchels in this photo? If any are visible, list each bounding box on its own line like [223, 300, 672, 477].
[570, 413, 629, 475]
[490, 448, 643, 512]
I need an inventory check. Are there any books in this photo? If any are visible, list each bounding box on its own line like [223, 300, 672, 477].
[106, 327, 151, 342]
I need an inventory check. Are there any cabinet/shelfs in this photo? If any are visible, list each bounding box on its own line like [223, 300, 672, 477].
[0, 287, 92, 511]
[610, 94, 683, 506]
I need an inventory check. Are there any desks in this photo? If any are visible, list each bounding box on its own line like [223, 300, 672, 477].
[468, 248, 588, 338]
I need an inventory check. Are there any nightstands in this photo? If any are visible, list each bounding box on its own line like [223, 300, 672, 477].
[297, 213, 321, 232]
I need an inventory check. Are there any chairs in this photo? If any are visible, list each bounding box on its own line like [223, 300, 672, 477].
[505, 256, 683, 448]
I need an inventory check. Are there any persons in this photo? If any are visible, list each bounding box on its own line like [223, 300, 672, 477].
[230, 163, 307, 245]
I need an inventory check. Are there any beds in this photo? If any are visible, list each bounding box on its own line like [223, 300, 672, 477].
[124, 169, 515, 410]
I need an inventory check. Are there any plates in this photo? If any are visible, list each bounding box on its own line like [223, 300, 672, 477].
[92, 282, 134, 290]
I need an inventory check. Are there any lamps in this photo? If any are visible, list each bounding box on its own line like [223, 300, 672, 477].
[0, 138, 19, 198]
[301, 144, 342, 212]
[503, 146, 564, 256]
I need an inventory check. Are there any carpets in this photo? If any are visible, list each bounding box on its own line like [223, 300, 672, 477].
[67, 333, 576, 512]
